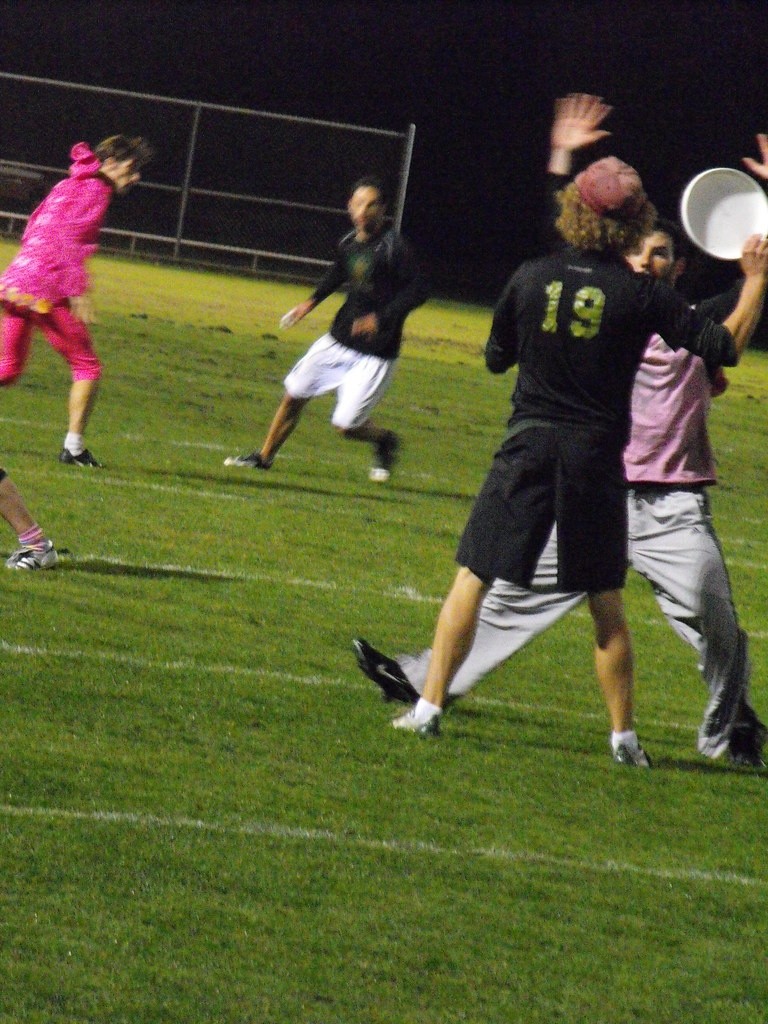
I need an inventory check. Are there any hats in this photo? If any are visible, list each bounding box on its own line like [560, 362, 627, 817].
[575, 157, 643, 219]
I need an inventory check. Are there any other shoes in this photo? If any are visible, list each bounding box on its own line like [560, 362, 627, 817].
[224, 453, 272, 470]
[370, 430, 398, 481]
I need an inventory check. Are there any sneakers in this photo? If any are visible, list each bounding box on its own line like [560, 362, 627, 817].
[613, 744, 653, 770]
[394, 710, 439, 739]
[59, 449, 97, 467]
[728, 722, 768, 772]
[5, 539, 58, 570]
[353, 637, 419, 705]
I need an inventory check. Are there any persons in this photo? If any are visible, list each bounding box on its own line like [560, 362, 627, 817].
[224, 174, 426, 482]
[350, 92, 767, 765]
[397, 157, 767, 765]
[0, 468, 59, 571]
[0, 134, 150, 467]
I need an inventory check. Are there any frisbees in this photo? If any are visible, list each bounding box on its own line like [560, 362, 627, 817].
[677, 166, 768, 262]
